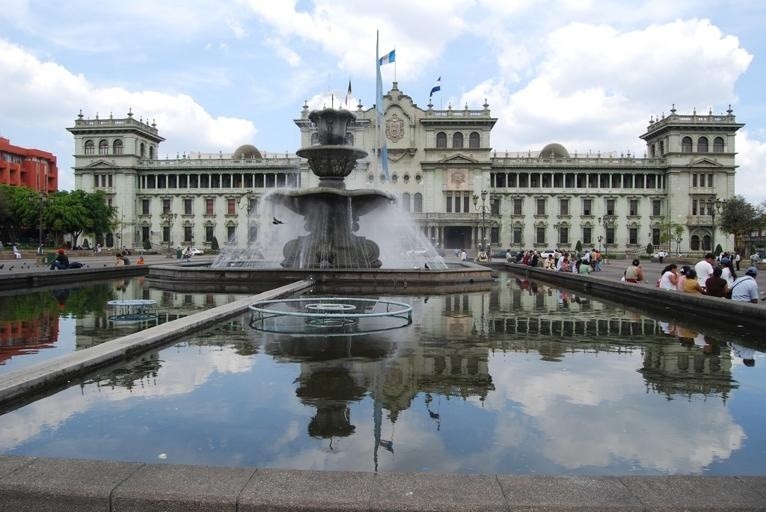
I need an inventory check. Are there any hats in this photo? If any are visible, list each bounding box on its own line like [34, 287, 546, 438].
[720, 257, 730, 264]
[746, 266, 758, 274]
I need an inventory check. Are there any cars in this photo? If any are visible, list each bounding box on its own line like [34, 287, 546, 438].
[408, 247, 429, 256]
[182, 247, 205, 257]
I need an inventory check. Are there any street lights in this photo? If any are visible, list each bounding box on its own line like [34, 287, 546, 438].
[707, 197, 727, 256]
[237, 188, 262, 253]
[29, 191, 54, 250]
[159, 210, 177, 254]
[473, 189, 496, 251]
[599, 214, 616, 256]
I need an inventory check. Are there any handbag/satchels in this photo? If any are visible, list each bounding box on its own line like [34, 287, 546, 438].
[726, 290, 733, 299]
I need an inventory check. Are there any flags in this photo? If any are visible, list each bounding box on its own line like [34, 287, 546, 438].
[378, 50, 394, 66]
[347, 80, 351, 94]
[429, 77, 440, 98]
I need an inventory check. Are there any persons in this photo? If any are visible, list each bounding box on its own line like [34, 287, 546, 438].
[180, 246, 191, 262]
[460, 248, 466, 261]
[657, 320, 755, 367]
[46, 249, 69, 270]
[12, 244, 21, 259]
[505, 248, 601, 275]
[116, 251, 130, 265]
[136, 256, 144, 264]
[622, 250, 761, 303]
[113, 253, 124, 266]
[505, 276, 589, 305]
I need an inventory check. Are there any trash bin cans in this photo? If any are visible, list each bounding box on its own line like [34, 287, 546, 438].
[176, 250, 181, 258]
[48, 252, 56, 262]
